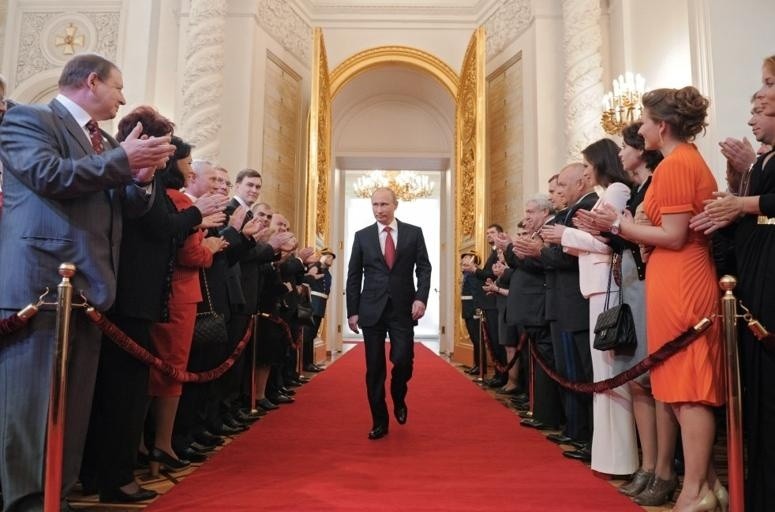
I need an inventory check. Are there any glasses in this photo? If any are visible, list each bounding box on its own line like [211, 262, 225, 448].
[218, 178, 233, 188]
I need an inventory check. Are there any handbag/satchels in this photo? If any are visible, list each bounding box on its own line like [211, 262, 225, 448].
[193, 311, 230, 346]
[593, 303, 637, 354]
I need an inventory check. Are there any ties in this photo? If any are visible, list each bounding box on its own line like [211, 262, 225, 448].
[86, 118, 104, 156]
[383, 227, 397, 272]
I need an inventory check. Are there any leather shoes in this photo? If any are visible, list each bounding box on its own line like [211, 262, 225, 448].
[171, 443, 208, 463]
[484, 376, 507, 388]
[546, 432, 577, 445]
[392, 392, 408, 426]
[284, 379, 303, 387]
[510, 393, 530, 411]
[562, 447, 593, 463]
[185, 440, 213, 454]
[367, 416, 392, 441]
[295, 373, 311, 384]
[255, 397, 281, 411]
[266, 388, 295, 405]
[223, 416, 250, 431]
[278, 385, 297, 396]
[463, 363, 488, 375]
[570, 439, 589, 448]
[519, 417, 562, 431]
[303, 363, 322, 373]
[496, 382, 521, 395]
[211, 422, 246, 436]
[195, 429, 227, 448]
[231, 408, 261, 425]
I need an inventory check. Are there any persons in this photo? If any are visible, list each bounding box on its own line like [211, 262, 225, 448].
[462, 57, 775, 512]
[0, 54, 335, 512]
[347, 188, 433, 441]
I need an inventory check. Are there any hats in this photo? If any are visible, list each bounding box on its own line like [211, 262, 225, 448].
[460, 249, 482, 266]
[320, 247, 336, 260]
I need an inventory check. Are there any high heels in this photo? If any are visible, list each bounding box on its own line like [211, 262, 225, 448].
[668, 490, 717, 512]
[711, 483, 730, 512]
[616, 469, 655, 498]
[98, 476, 158, 504]
[148, 445, 191, 477]
[633, 472, 680, 507]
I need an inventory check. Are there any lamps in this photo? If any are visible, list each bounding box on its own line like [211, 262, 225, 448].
[600, 72, 647, 138]
[352, 170, 435, 201]
